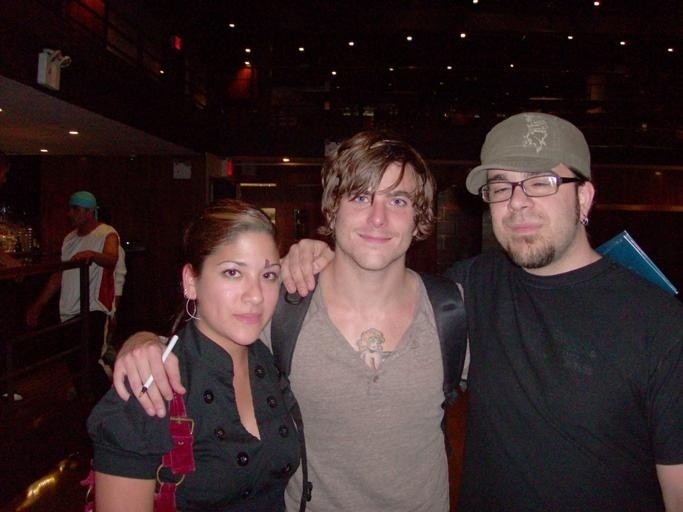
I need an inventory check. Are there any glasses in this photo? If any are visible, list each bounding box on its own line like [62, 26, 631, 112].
[479, 172, 589, 204]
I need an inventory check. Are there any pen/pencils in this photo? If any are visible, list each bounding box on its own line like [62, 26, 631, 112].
[137, 334, 179, 400]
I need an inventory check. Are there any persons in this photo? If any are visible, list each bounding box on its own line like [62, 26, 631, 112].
[279, 113, 682, 510]
[23, 190, 119, 392]
[112, 130, 472, 512]
[85, 199, 301, 509]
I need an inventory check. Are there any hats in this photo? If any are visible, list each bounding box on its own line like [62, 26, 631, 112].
[466, 111, 592, 194]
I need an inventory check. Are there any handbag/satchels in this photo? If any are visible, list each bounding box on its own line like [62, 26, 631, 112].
[19, 448, 187, 511]
